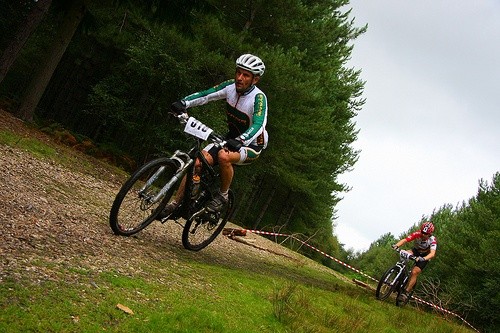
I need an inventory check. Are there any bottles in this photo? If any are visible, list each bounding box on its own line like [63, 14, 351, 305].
[191, 173, 200, 201]
[193, 189, 207, 207]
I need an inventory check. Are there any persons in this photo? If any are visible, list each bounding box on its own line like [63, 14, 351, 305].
[392, 222, 438, 302]
[151, 54, 269, 220]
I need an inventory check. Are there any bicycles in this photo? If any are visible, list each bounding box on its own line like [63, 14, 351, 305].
[375, 244, 420, 308]
[109, 106, 236, 251]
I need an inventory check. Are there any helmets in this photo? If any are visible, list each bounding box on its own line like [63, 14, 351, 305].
[421, 222, 435, 233]
[235, 54, 265, 77]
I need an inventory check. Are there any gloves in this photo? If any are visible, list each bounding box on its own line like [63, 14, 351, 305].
[392, 245, 398, 250]
[223, 139, 243, 152]
[416, 256, 425, 262]
[171, 102, 185, 113]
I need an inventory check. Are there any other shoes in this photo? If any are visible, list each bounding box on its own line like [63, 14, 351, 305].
[204, 193, 229, 220]
[151, 204, 176, 220]
[401, 291, 409, 298]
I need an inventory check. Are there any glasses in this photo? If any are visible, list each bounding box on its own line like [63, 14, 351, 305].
[422, 231, 429, 235]
